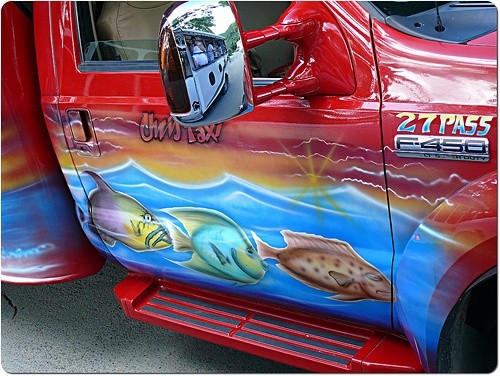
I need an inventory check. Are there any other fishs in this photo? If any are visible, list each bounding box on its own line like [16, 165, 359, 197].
[249, 229, 396, 302]
[159, 207, 268, 288]
[80, 170, 173, 252]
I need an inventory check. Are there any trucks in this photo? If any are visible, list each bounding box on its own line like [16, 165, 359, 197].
[1, 0, 498, 376]
